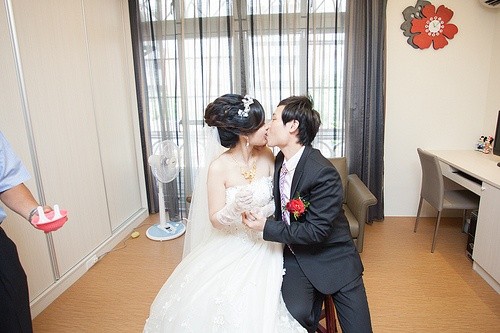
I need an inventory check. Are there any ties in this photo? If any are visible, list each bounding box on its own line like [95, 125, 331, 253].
[279, 164, 295, 255]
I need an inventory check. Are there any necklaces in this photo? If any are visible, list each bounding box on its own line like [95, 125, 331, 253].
[229, 150, 258, 184]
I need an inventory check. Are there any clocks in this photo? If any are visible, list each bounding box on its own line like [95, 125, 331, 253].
[400, 0, 458, 51]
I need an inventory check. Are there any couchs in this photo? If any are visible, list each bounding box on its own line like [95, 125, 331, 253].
[325, 157, 377, 252]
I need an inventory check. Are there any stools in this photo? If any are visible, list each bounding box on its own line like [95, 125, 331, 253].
[317, 294, 340, 333]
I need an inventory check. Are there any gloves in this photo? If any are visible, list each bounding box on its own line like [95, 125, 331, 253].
[216, 188, 256, 225]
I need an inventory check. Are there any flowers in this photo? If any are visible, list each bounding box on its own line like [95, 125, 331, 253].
[286, 195, 306, 219]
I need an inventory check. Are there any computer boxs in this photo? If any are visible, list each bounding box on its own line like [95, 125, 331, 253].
[466, 211, 478, 264]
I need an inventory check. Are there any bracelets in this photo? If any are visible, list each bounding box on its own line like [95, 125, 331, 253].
[28, 209, 38, 224]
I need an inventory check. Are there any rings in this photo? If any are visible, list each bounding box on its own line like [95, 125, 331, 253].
[245, 224, 247, 226]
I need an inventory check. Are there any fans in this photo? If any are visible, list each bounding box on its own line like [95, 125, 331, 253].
[142, 140, 184, 242]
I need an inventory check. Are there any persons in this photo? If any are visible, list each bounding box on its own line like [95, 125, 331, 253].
[0, 129, 53, 333]
[476, 136, 494, 154]
[142, 96, 309, 333]
[242, 97, 373, 333]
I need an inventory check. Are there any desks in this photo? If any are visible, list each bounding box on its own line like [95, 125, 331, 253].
[426, 145, 500, 294]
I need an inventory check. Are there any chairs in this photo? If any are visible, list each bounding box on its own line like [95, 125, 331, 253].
[414, 148, 479, 253]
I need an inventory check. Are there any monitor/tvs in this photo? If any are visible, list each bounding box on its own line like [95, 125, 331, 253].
[493, 111, 500, 156]
